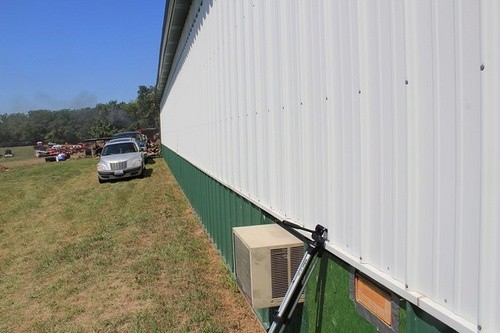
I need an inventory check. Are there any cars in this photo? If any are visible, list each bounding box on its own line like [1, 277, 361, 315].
[96, 137, 146, 183]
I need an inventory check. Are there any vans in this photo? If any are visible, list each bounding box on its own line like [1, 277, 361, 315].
[114, 131, 148, 162]
